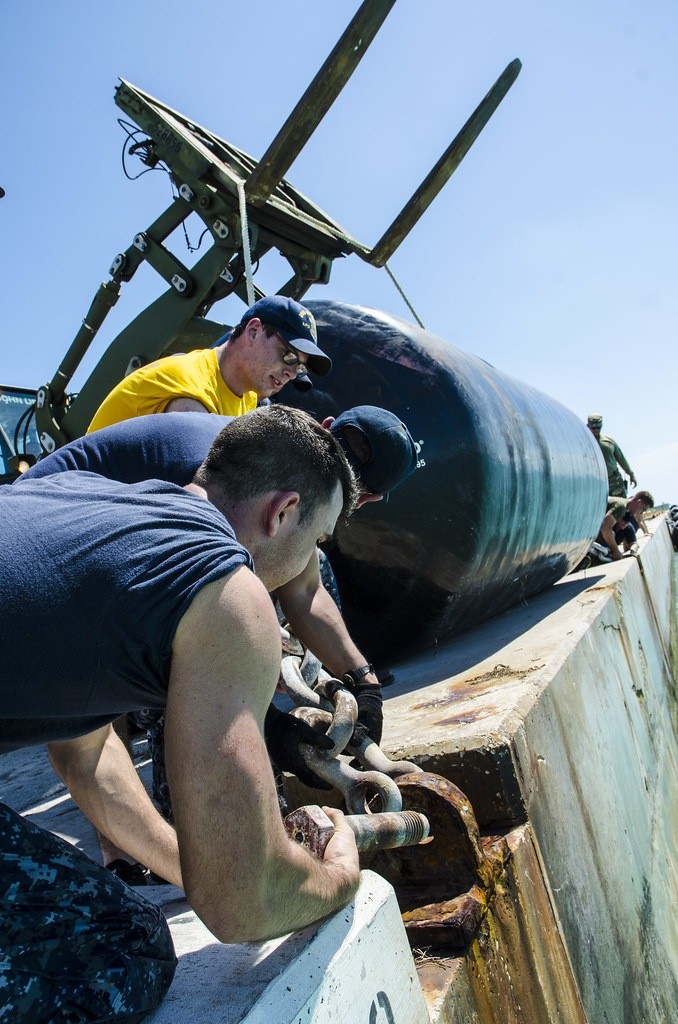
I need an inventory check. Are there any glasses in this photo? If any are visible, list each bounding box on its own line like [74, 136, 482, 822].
[263, 322, 308, 377]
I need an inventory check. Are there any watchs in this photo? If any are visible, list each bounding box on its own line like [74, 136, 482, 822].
[344, 662, 375, 687]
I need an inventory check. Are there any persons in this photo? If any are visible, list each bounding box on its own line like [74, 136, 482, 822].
[85, 293, 318, 438]
[586, 415, 656, 561]
[0, 404, 418, 1021]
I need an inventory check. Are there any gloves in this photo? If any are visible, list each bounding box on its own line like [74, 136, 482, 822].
[263, 703, 336, 790]
[340, 683, 383, 767]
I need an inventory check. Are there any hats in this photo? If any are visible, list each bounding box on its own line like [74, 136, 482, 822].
[587, 414, 602, 428]
[328, 405, 420, 502]
[235, 294, 332, 377]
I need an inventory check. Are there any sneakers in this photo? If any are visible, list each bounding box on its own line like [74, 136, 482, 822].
[102, 859, 186, 910]
